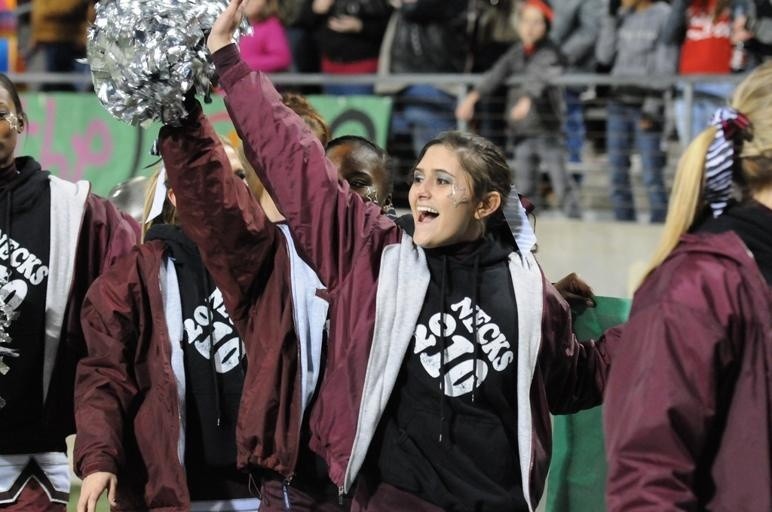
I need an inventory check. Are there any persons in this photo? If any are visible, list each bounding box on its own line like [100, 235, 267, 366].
[1, 0, 772, 510]
